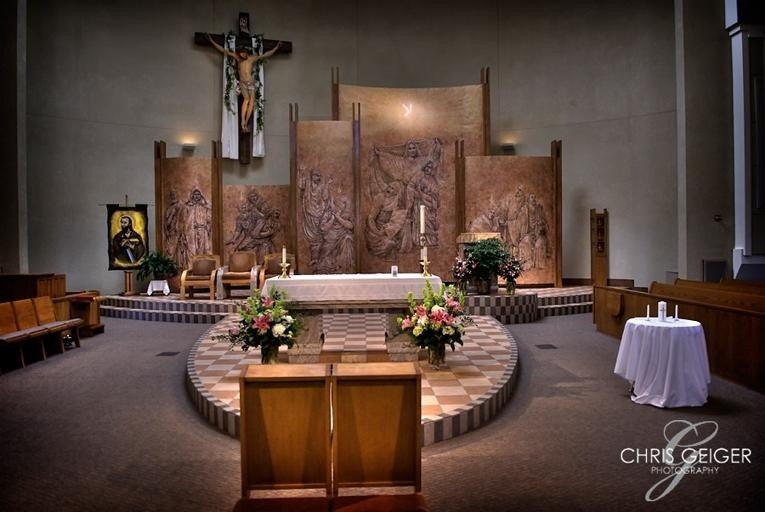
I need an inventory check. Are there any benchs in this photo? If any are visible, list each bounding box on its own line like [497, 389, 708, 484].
[591, 274, 765, 397]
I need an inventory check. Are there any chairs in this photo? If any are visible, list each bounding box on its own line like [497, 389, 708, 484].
[219, 251, 261, 299]
[0, 295, 85, 371]
[259, 253, 296, 291]
[179, 254, 221, 300]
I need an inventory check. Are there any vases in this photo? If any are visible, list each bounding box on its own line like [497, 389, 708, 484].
[476, 276, 491, 295]
[430, 343, 446, 368]
[263, 340, 288, 364]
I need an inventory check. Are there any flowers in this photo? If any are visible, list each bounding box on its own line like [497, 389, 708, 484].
[451, 237, 523, 294]
[394, 278, 478, 351]
[210, 282, 310, 355]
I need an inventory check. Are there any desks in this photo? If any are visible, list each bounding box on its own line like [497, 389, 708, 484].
[262, 273, 445, 300]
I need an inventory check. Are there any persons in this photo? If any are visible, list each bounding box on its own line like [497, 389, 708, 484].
[225, 189, 281, 265]
[365, 138, 439, 258]
[113, 216, 145, 264]
[298, 168, 354, 273]
[208, 33, 281, 133]
[166, 187, 211, 270]
[468, 185, 553, 271]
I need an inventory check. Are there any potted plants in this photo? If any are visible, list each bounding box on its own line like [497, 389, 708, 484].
[135, 251, 178, 280]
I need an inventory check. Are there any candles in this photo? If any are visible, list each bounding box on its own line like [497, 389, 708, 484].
[421, 249, 424, 261]
[646, 304, 651, 318]
[423, 246, 428, 261]
[675, 305, 679, 318]
[282, 244, 286, 264]
[420, 205, 426, 234]
